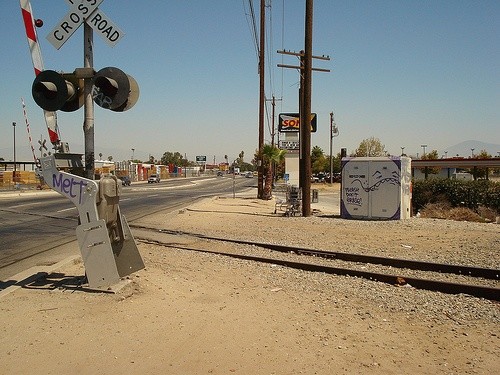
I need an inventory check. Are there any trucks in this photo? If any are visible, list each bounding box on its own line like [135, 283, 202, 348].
[234, 167, 240, 175]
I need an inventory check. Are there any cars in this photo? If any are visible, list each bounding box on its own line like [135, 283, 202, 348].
[240, 171, 258, 178]
[118, 176, 131, 186]
[310, 172, 341, 182]
[217, 172, 223, 176]
[148, 174, 160, 183]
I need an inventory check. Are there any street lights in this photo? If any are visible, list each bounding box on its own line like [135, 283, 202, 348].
[12, 121, 16, 171]
[131, 148, 135, 163]
[421, 145, 428, 154]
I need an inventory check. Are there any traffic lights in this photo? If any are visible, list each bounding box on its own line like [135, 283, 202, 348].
[31, 67, 139, 112]
